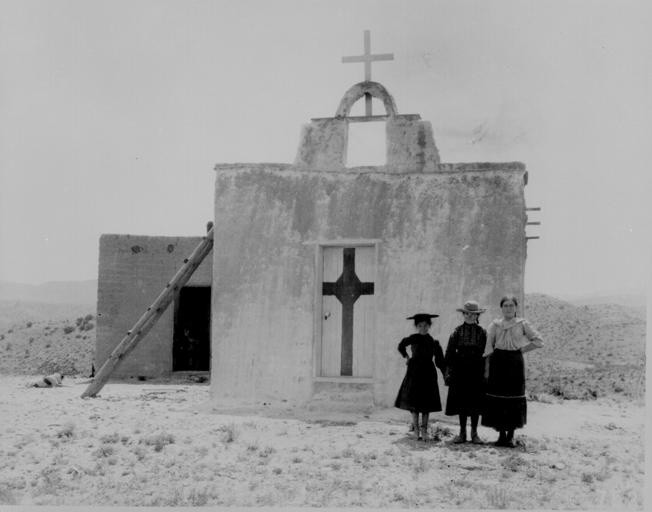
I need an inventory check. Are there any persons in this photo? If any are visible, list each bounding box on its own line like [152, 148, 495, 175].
[394, 310, 447, 440]
[482, 293, 545, 449]
[444, 299, 495, 444]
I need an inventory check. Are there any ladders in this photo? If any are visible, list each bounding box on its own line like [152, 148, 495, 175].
[81, 220, 214, 396]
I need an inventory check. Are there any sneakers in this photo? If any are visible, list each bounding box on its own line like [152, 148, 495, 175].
[470, 431, 486, 445]
[492, 435, 518, 449]
[452, 430, 469, 444]
[406, 428, 437, 443]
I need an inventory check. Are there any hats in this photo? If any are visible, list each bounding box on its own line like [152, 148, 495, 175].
[405, 313, 440, 321]
[455, 301, 487, 315]
